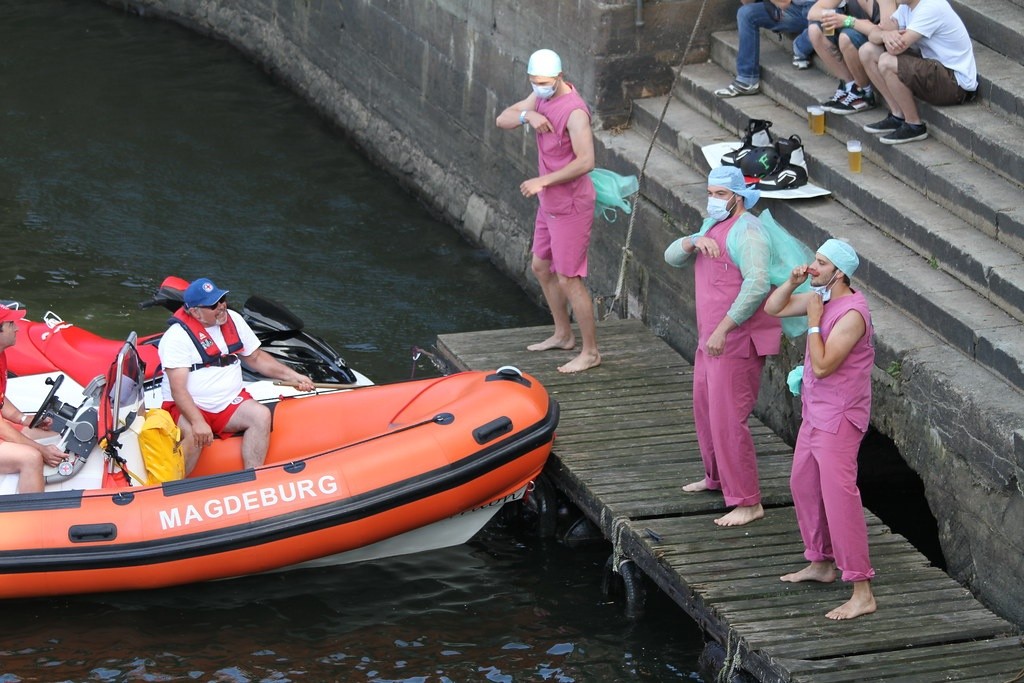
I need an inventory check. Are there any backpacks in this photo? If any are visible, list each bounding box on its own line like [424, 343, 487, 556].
[110, 408, 185, 487]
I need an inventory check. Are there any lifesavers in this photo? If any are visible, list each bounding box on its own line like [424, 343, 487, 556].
[523, 476, 558, 537]
[598, 554, 652, 616]
[696, 640, 749, 682]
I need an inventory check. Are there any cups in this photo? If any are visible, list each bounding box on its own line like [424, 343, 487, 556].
[822, 8, 834, 37]
[846, 139, 862, 173]
[807, 105, 820, 131]
[811, 109, 825, 136]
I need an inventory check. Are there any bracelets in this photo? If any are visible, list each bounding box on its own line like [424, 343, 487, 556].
[691, 235, 698, 246]
[20, 415, 28, 425]
[843, 16, 856, 29]
[807, 326, 820, 335]
[519, 110, 528, 124]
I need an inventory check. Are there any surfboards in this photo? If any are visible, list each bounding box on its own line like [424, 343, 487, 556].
[700, 141, 832, 200]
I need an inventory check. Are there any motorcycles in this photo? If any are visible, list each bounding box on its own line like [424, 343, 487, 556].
[0, 274, 376, 412]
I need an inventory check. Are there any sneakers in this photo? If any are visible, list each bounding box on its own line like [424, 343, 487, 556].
[792, 55, 810, 70]
[714, 81, 760, 98]
[863, 112, 904, 133]
[755, 138, 809, 190]
[720, 119, 772, 168]
[820, 79, 876, 115]
[880, 121, 928, 144]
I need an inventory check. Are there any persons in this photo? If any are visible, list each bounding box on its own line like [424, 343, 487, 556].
[496, 48, 602, 374]
[0, 303, 70, 495]
[663, 165, 783, 528]
[763, 238, 878, 621]
[157, 277, 316, 485]
[713, 0, 980, 145]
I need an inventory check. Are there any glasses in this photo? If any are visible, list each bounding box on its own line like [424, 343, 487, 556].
[201, 294, 226, 310]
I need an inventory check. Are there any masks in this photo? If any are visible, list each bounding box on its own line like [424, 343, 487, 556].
[531, 80, 559, 100]
[810, 270, 840, 302]
[707, 193, 737, 221]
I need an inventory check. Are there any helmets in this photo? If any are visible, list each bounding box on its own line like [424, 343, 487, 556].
[740, 147, 779, 178]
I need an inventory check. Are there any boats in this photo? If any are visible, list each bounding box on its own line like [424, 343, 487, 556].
[0, 367, 561, 603]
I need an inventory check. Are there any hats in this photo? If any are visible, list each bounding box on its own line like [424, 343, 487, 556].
[183, 278, 229, 307]
[0, 303, 27, 324]
